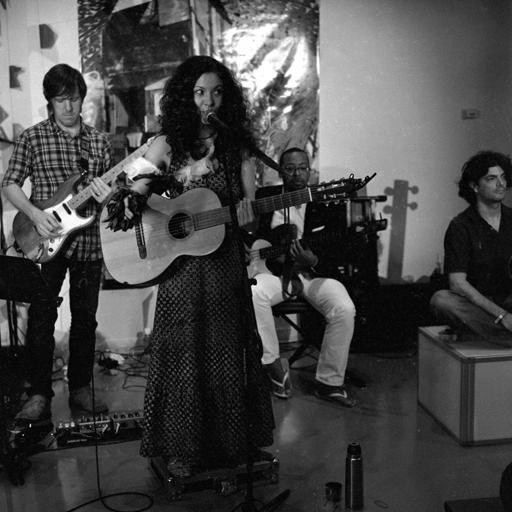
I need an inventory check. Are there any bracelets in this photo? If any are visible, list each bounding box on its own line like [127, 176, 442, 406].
[494, 310, 507, 324]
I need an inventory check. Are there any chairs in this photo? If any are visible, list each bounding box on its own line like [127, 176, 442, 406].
[254, 186, 367, 389]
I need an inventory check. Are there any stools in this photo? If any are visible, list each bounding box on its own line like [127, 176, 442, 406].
[417, 323, 512, 446]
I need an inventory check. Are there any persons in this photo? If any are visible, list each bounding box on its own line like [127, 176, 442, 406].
[429, 151, 512, 348]
[7, 63, 113, 423]
[243, 147, 359, 409]
[115, 54, 276, 479]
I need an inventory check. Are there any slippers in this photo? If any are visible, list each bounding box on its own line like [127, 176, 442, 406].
[266, 368, 292, 398]
[314, 388, 359, 408]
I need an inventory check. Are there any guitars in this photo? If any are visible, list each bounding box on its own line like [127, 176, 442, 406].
[13, 136, 155, 262]
[241, 213, 387, 278]
[100, 177, 351, 285]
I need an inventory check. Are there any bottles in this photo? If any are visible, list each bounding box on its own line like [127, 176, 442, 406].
[345, 443, 363, 510]
[144, 328, 152, 346]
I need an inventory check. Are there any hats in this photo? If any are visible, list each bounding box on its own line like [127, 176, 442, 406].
[283, 164, 309, 173]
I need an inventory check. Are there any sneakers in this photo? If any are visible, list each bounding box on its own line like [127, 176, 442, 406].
[68, 384, 109, 413]
[15, 394, 51, 422]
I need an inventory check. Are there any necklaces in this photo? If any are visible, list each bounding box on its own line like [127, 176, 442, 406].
[197, 128, 218, 140]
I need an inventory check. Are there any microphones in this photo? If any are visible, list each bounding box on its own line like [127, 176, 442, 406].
[205, 108, 253, 154]
